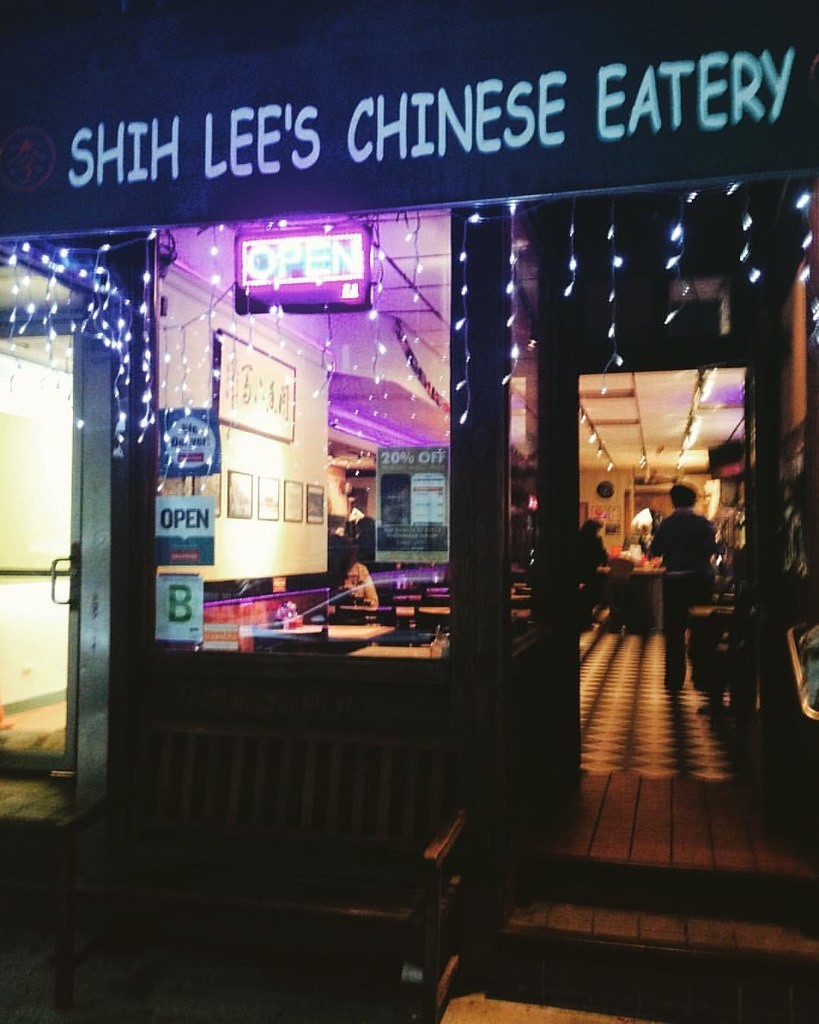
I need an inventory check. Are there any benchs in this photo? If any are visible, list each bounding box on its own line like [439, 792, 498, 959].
[53, 716, 478, 1024]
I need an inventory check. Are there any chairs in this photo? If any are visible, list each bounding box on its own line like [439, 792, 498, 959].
[254, 627, 326, 655]
[335, 602, 397, 627]
[414, 605, 449, 628]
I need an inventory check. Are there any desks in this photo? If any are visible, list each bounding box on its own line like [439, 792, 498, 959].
[262, 587, 450, 659]
[601, 566, 666, 633]
[690, 605, 738, 719]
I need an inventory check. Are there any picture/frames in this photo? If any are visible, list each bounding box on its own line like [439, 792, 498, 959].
[227, 470, 255, 519]
[257, 477, 281, 520]
[214, 328, 296, 445]
[306, 484, 324, 525]
[193, 466, 221, 518]
[283, 480, 304, 522]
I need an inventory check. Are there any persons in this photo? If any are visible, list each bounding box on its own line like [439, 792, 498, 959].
[579, 517, 608, 631]
[334, 538, 379, 609]
[650, 484, 716, 692]
